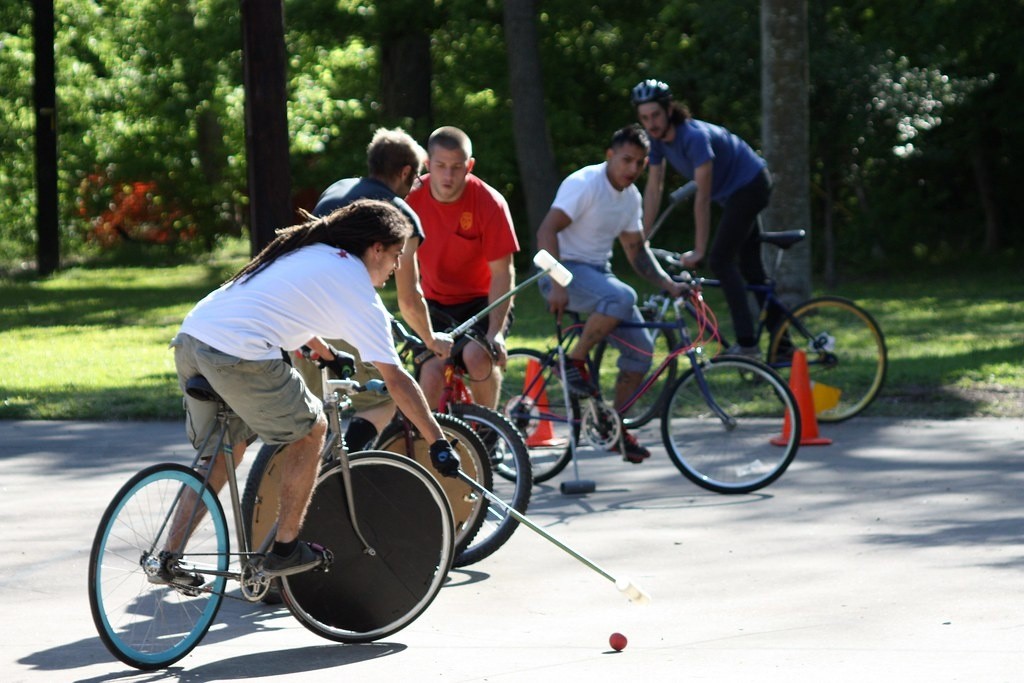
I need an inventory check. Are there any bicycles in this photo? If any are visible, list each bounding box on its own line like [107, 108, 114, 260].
[490, 275, 801, 496]
[624, 227, 888, 429]
[88, 363, 456, 671]
[238, 319, 535, 608]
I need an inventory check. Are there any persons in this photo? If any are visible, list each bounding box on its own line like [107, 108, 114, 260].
[282, 127, 456, 454]
[403, 126, 521, 414]
[148, 197, 463, 585]
[633, 79, 802, 368]
[537, 123, 692, 460]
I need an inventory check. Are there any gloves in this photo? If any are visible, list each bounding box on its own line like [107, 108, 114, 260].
[317, 346, 357, 378]
[428, 438, 462, 478]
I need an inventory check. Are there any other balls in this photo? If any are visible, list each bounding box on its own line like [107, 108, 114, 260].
[609, 632, 627, 652]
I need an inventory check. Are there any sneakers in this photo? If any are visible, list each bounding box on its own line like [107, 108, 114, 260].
[263, 541, 322, 576]
[600, 424, 650, 459]
[148, 553, 206, 586]
[552, 355, 599, 397]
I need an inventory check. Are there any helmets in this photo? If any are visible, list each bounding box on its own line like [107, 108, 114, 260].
[632, 79, 671, 103]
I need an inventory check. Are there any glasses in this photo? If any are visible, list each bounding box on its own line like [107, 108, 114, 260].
[410, 173, 423, 192]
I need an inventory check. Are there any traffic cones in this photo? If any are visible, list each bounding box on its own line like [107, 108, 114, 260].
[520, 357, 570, 446]
[769, 352, 835, 445]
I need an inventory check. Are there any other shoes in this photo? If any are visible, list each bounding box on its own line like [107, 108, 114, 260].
[475, 424, 503, 464]
[721, 343, 762, 360]
[769, 347, 798, 363]
